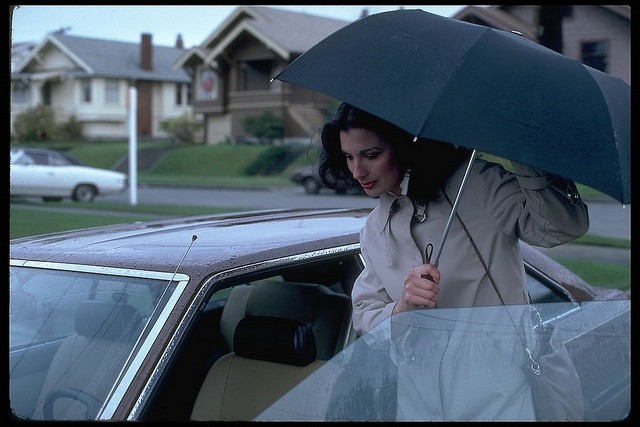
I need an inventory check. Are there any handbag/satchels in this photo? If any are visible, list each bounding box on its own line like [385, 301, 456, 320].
[522, 306, 585, 424]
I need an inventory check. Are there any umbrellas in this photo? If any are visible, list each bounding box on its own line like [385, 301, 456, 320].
[270, 10, 631, 284]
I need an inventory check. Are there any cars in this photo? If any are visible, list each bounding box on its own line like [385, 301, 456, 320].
[10, 148, 127, 202]
[293, 165, 330, 194]
[10, 209, 627, 422]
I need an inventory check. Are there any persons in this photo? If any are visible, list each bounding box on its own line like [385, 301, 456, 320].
[317, 103, 592, 421]
